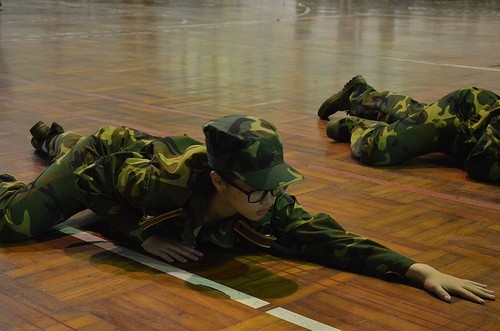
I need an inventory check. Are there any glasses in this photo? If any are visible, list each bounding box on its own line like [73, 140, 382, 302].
[228, 181, 288, 203]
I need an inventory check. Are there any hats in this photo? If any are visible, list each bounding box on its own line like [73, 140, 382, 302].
[202, 114, 304, 190]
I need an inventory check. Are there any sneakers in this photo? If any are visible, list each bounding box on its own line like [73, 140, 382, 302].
[316, 75, 367, 119]
[327, 115, 353, 141]
[29, 120, 50, 149]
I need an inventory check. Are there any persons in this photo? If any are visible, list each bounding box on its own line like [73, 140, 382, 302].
[317, 74, 500, 186]
[0, 115, 495, 305]
